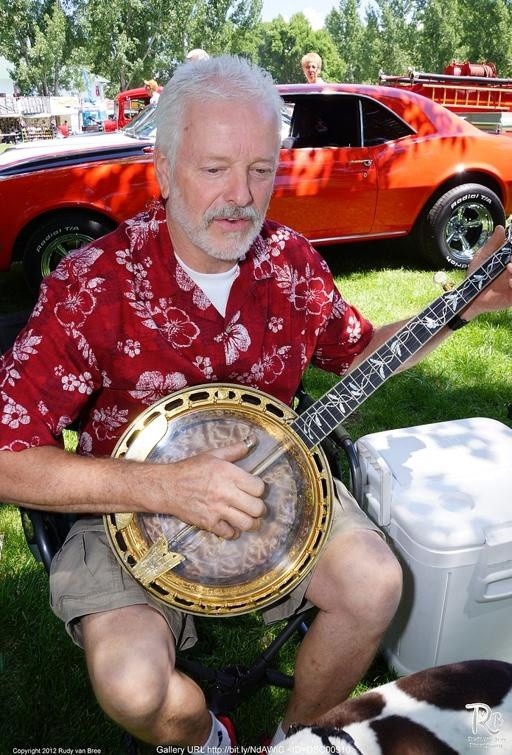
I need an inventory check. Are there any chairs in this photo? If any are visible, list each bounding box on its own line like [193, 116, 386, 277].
[0, 309, 363, 723]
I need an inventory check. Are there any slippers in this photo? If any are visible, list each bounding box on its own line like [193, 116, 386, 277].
[259, 738, 273, 755]
[215, 714, 238, 755]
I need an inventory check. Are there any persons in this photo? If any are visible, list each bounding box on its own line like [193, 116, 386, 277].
[62, 121, 68, 126]
[186, 49, 209, 62]
[302, 52, 326, 84]
[144, 80, 160, 103]
[0, 54, 512, 755]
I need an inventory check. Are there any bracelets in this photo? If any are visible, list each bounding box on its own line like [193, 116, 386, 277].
[447, 315, 470, 331]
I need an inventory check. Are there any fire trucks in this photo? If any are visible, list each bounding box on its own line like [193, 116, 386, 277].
[379, 60, 512, 135]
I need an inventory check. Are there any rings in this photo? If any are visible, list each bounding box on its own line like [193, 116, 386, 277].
[243, 437, 255, 450]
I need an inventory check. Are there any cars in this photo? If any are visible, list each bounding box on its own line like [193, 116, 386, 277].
[0, 83, 512, 305]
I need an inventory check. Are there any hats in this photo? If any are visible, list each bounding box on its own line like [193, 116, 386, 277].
[143, 78, 159, 91]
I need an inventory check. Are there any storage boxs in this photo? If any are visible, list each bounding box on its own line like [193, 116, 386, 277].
[352, 417, 511, 677]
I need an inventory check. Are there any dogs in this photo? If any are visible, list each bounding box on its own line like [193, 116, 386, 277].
[263, 658, 512, 755]
